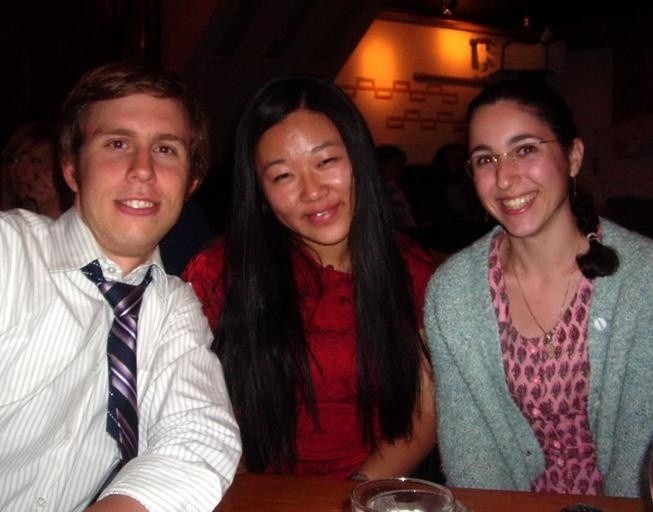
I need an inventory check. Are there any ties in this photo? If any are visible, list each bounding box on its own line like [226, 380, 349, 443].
[81, 261, 153, 507]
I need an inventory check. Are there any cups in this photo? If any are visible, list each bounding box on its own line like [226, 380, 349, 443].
[347, 476, 468, 512]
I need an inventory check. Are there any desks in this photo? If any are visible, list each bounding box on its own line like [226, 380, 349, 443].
[216, 473, 653, 512]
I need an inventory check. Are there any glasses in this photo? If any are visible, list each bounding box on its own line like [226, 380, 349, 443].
[464, 140, 561, 182]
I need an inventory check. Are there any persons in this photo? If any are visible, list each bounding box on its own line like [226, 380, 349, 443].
[0, 57, 244, 512]
[175, 74, 443, 484]
[423, 72, 652, 498]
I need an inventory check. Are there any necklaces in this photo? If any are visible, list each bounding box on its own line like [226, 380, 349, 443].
[506, 245, 582, 341]
